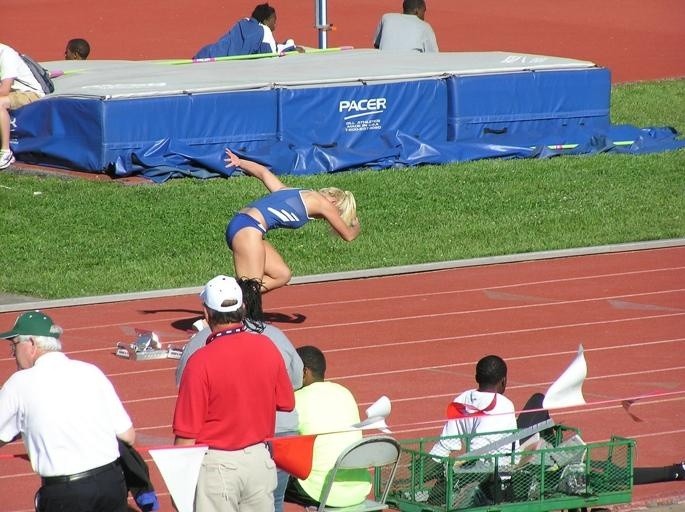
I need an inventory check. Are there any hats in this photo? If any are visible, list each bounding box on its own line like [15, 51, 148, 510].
[0, 309, 59, 339]
[199, 274, 243, 313]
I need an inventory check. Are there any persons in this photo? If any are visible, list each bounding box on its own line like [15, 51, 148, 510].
[193, 3, 297, 59]
[284, 346, 373, 508]
[192, 147, 361, 331]
[171, 277, 303, 512]
[374, 0, 438, 54]
[0, 43, 46, 170]
[0, 311, 135, 512]
[63, 38, 91, 60]
[413, 354, 554, 481]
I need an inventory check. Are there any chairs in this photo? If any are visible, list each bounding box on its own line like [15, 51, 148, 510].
[306, 436, 402, 512]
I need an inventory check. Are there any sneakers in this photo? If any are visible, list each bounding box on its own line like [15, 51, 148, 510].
[0, 149, 16, 170]
[192, 319, 204, 333]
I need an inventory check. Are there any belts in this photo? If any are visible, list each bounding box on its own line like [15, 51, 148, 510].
[42, 457, 122, 486]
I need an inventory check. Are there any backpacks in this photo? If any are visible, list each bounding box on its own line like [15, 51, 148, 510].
[14, 54, 55, 96]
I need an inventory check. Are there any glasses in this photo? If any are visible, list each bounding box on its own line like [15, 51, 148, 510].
[10, 341, 19, 354]
